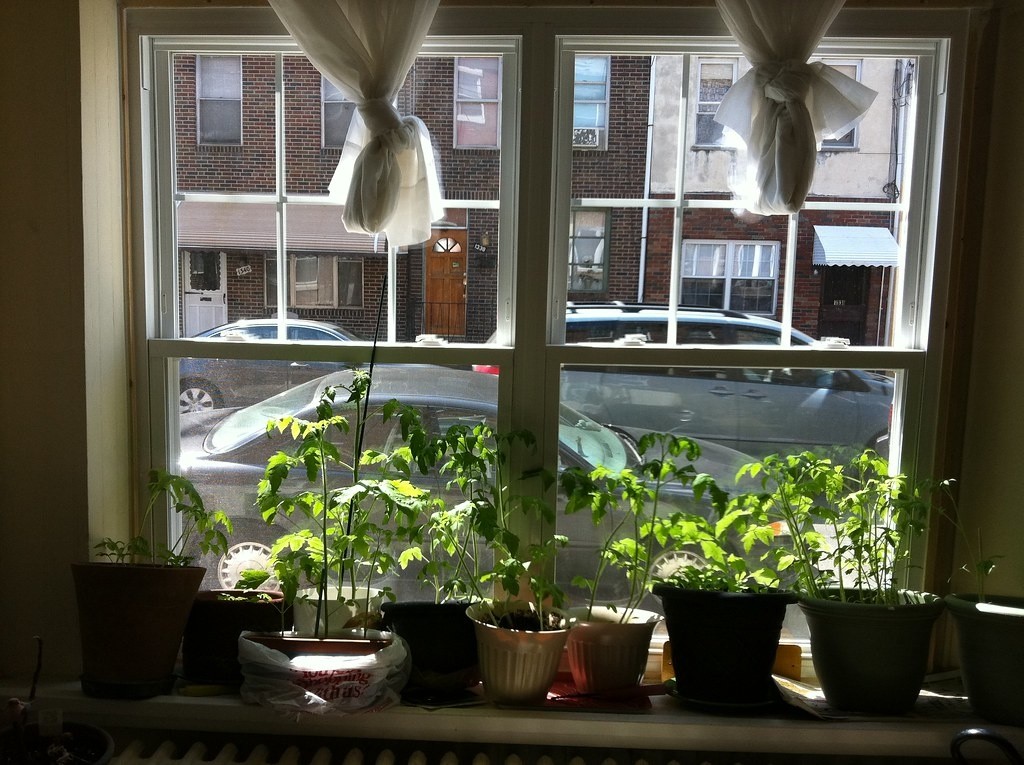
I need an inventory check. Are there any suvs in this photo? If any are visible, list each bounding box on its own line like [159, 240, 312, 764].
[470, 301, 895, 524]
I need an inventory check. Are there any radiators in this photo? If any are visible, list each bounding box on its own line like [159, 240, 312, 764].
[107, 739, 742, 765]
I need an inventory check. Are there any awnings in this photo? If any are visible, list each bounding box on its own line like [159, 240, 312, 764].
[813, 225, 900, 267]
[178, 202, 409, 255]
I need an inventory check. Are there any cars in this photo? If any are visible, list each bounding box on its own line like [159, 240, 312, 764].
[180, 364, 820, 622]
[180, 317, 373, 416]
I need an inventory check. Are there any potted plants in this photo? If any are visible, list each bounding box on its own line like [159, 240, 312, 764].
[70, 275, 1024, 725]
